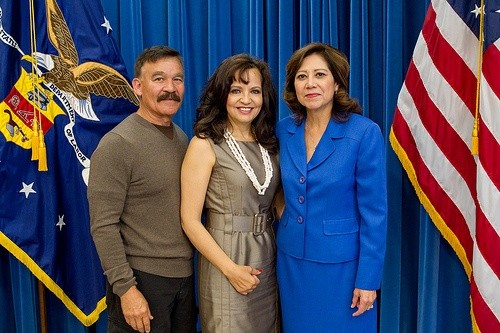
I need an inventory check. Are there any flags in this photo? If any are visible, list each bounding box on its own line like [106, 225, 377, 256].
[0, 0, 142, 328]
[388, 0, 500, 333]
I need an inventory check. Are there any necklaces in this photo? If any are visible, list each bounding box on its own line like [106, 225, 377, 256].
[223, 125, 273, 195]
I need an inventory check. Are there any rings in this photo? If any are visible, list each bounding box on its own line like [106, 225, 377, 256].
[370, 304, 373, 309]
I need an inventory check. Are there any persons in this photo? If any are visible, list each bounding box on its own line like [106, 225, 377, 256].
[274, 42, 387, 333]
[180, 53, 281, 333]
[86, 47, 198, 333]
